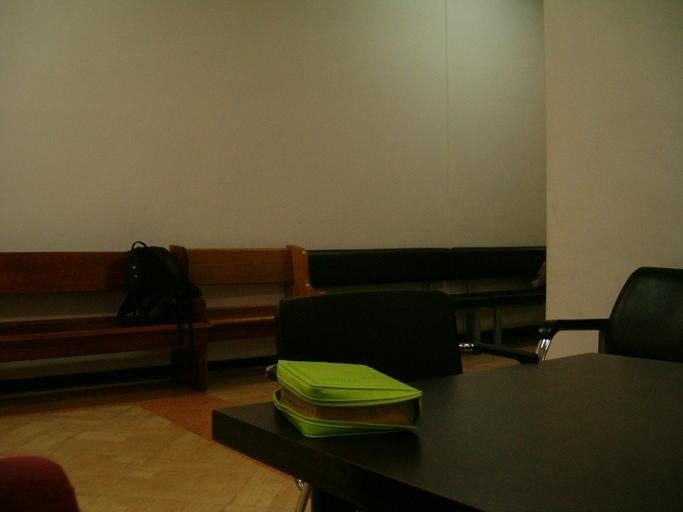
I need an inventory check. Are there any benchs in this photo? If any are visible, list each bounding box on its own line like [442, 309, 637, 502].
[1, 241, 306, 391]
[307, 245, 547, 356]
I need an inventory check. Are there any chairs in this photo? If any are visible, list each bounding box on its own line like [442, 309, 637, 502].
[535, 264, 681, 366]
[275, 293, 464, 377]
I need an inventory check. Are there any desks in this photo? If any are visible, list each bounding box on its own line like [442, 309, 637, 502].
[211, 348, 682, 512]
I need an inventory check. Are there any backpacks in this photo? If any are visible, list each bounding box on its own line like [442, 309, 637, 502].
[115, 241, 202, 331]
